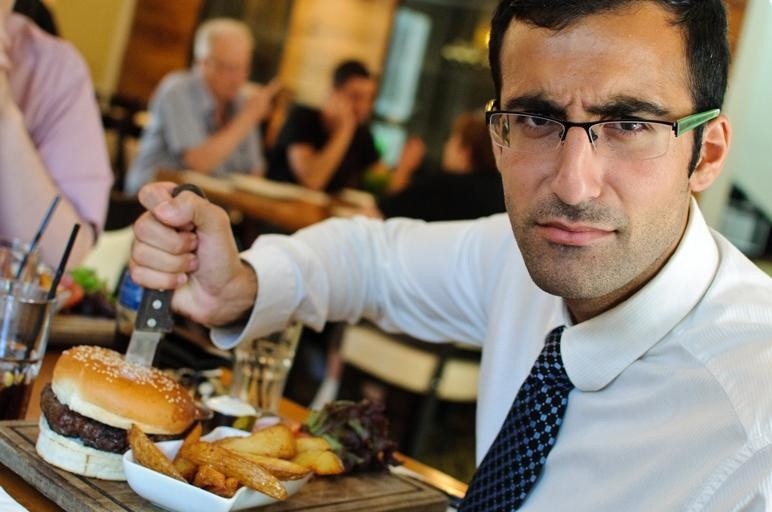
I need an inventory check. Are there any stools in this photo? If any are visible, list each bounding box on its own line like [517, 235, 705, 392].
[338, 318, 482, 459]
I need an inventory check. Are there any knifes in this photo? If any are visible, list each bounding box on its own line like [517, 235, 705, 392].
[120, 182, 199, 380]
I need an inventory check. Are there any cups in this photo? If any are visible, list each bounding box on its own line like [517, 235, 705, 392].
[2, 284, 60, 422]
[233, 309, 304, 419]
[1, 238, 54, 285]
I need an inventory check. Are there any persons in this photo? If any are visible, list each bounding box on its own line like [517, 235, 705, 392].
[125, 16, 299, 233]
[127, 2, 771, 512]
[377, 99, 504, 221]
[271, 51, 379, 195]
[0, 1, 116, 274]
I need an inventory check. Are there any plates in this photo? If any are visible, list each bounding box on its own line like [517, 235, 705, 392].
[176, 169, 380, 222]
[120, 426, 318, 510]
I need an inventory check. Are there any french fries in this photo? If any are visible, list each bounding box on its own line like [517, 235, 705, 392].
[123, 417, 345, 506]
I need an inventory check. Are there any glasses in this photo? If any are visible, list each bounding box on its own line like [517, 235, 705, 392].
[484, 101, 720, 161]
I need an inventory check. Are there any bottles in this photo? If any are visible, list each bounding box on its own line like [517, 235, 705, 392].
[113, 265, 150, 353]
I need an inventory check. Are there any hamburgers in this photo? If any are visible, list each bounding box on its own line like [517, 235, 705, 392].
[35, 343, 197, 484]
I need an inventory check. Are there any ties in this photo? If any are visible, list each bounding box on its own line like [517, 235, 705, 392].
[459, 326, 575, 512]
